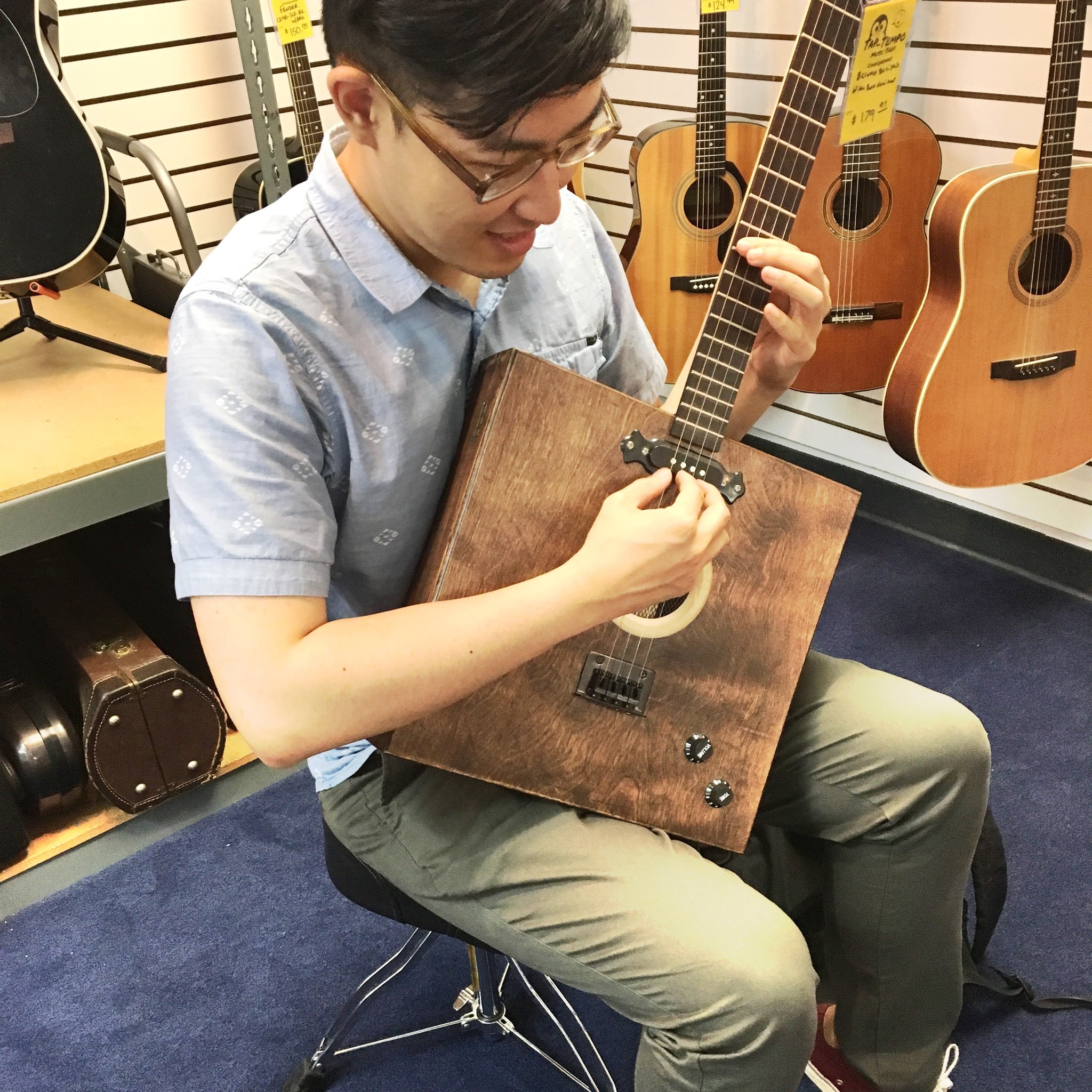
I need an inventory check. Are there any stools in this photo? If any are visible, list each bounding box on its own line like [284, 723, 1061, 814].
[287, 818, 618, 1092]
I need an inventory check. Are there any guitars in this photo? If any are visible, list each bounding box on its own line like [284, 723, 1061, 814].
[230, 0, 341, 220]
[749, 0, 929, 394]
[882, 2, 1090, 488]
[0, 0, 125, 299]
[614, 1, 769, 384]
[375, 0, 919, 852]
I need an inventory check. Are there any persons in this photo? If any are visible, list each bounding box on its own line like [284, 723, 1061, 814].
[164, 0, 992, 1092]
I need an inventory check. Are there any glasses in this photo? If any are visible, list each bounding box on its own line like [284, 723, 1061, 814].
[357, 60, 617, 203]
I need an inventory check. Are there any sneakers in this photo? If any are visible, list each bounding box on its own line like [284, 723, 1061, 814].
[805, 1002, 960, 1092]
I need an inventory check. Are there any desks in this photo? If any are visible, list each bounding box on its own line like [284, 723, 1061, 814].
[0, 282, 169, 557]
